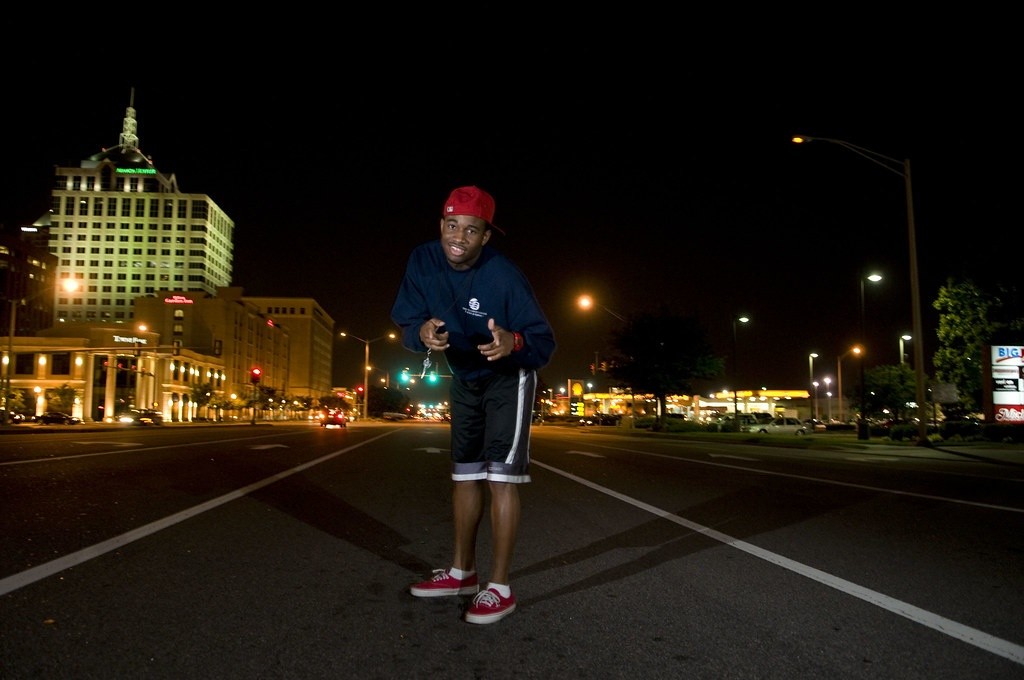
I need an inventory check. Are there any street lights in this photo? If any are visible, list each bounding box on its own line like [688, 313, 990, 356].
[809, 353, 818, 430]
[733, 317, 749, 432]
[859, 271, 882, 314]
[899, 334, 913, 363]
[339, 330, 396, 419]
[4, 278, 78, 425]
[790, 135, 929, 447]
[837, 347, 861, 421]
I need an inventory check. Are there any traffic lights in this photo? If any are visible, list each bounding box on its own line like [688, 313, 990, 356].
[402, 369, 408, 381]
[250, 368, 261, 384]
[430, 371, 435, 382]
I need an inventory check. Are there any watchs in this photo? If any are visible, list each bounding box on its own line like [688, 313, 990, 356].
[513, 331, 524, 352]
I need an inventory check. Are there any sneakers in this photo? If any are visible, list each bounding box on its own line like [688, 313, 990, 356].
[410, 568, 479, 597]
[465, 585, 517, 624]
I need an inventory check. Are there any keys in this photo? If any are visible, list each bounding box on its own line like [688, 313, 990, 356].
[420, 322, 448, 379]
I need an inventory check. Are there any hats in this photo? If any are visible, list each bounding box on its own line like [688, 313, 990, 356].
[443, 186, 506, 237]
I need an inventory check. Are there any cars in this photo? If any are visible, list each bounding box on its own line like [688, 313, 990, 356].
[115, 407, 163, 426]
[319, 410, 347, 428]
[0, 409, 26, 426]
[32, 412, 81, 426]
[530, 408, 977, 437]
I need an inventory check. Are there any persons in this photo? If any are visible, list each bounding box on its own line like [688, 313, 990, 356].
[387, 185, 556, 625]
